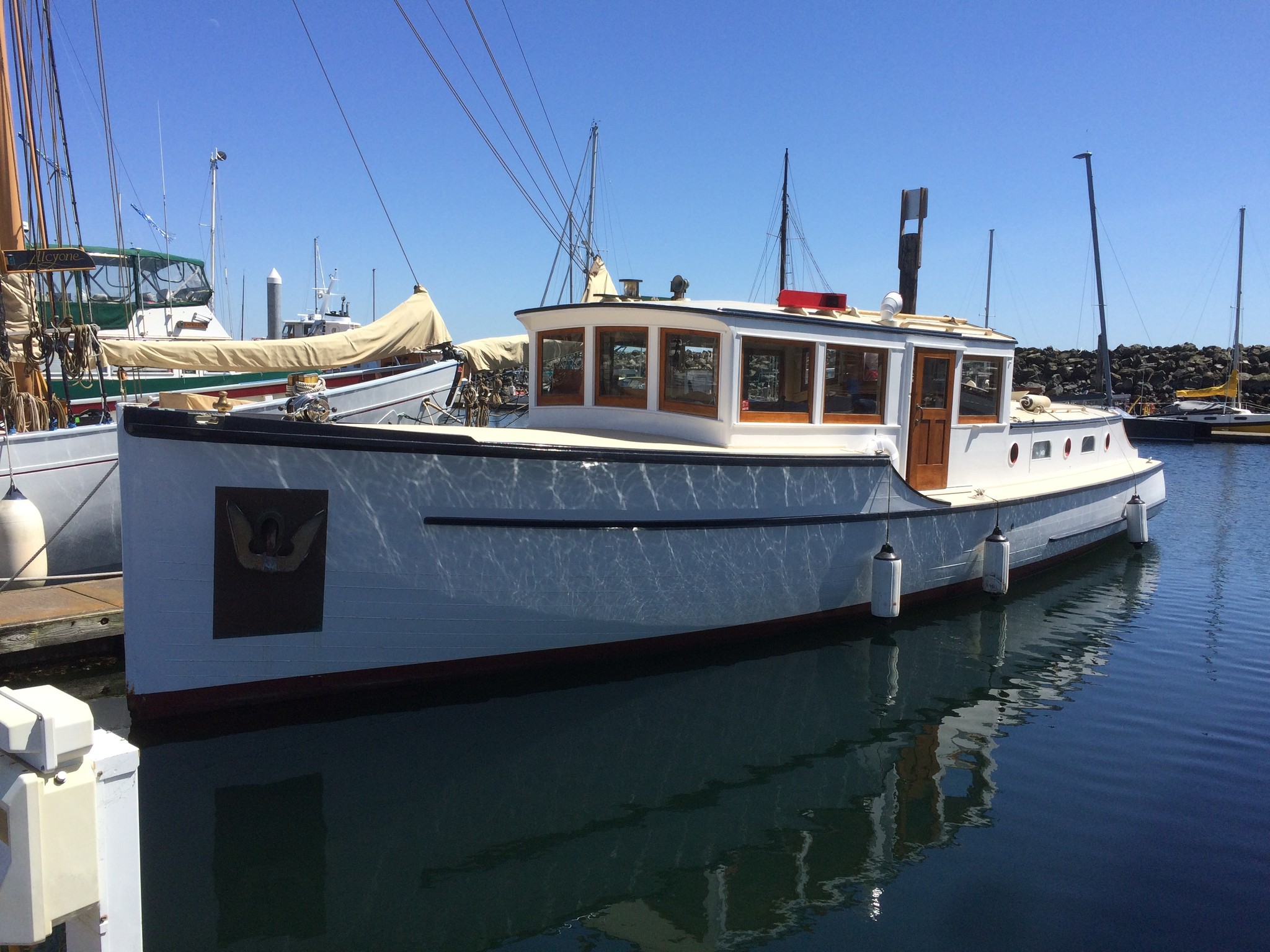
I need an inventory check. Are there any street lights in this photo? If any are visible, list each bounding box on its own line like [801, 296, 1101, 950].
[1073, 152, 1111, 406]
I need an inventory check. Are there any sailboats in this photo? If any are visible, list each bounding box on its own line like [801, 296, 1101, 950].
[1052, 206, 1270, 433]
[0, 4, 641, 581]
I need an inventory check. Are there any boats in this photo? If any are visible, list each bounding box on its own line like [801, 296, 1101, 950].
[115, 298, 1166, 729]
[250, 235, 443, 373]
[23, 244, 327, 399]
